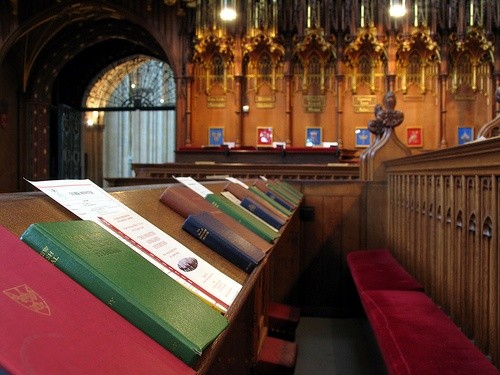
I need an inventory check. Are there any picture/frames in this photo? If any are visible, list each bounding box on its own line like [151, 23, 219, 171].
[355, 127, 372, 148]
[456, 126, 474, 144]
[405, 127, 424, 148]
[256, 126, 273, 147]
[208, 127, 224, 146]
[304, 126, 322, 147]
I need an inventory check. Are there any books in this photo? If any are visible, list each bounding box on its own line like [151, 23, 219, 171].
[20, 220, 228, 363]
[159, 187, 274, 253]
[0, 223, 198, 374]
[205, 177, 304, 242]
[181, 212, 266, 271]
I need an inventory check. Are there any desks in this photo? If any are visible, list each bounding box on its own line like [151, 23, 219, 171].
[0, 177, 304, 374]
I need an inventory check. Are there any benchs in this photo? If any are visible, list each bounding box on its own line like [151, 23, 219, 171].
[347, 248, 425, 294]
[359, 289, 500, 375]
